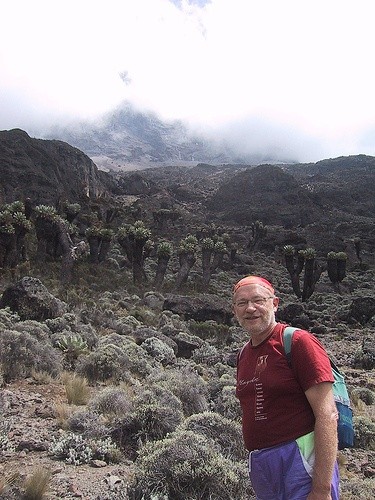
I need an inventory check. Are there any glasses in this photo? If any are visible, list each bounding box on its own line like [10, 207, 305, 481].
[234, 297, 274, 308]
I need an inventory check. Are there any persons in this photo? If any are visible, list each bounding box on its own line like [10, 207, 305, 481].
[232, 275, 339, 500]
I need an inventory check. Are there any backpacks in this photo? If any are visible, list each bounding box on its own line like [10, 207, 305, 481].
[238, 327, 354, 450]
